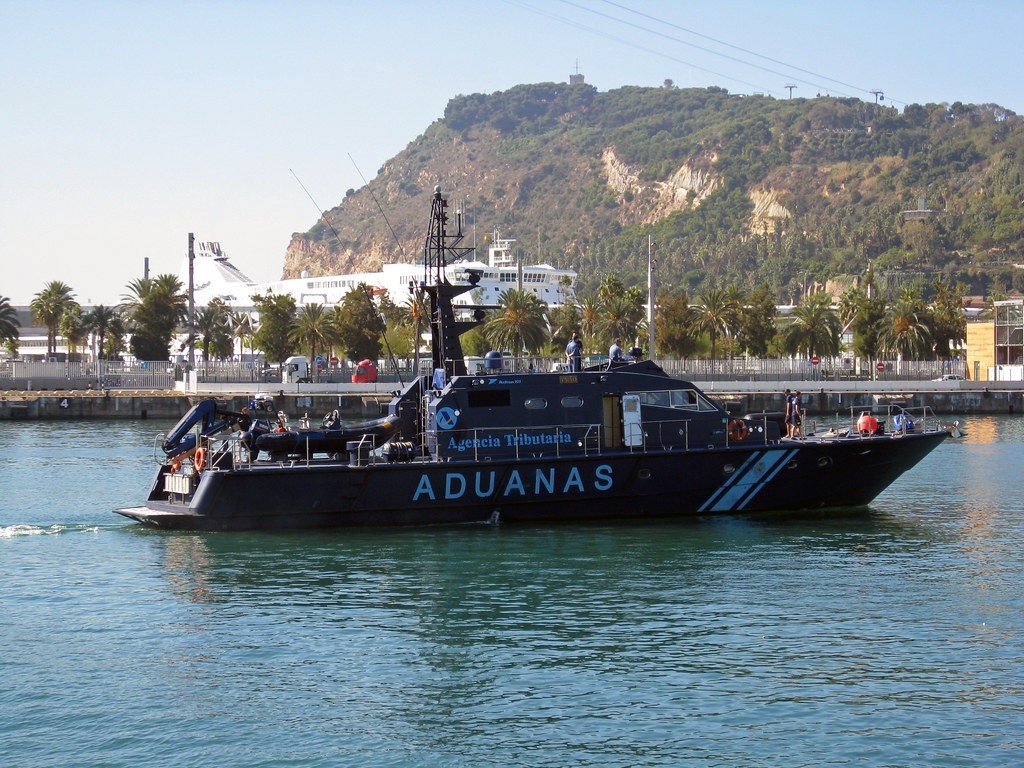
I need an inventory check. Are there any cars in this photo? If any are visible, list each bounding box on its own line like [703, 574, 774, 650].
[930, 374, 965, 383]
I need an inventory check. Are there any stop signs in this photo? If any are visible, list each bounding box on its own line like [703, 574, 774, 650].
[876, 363, 885, 372]
[811, 357, 819, 365]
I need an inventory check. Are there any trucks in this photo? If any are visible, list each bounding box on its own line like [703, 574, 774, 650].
[281, 356, 311, 383]
[351, 359, 378, 383]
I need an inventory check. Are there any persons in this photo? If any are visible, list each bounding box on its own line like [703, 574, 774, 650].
[565, 334, 580, 372]
[791, 391, 807, 440]
[784, 388, 797, 438]
[263, 361, 272, 377]
[568, 332, 583, 372]
[608, 338, 626, 366]
[216, 405, 296, 464]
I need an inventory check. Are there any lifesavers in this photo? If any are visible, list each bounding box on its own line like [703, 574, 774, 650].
[728, 419, 747, 441]
[194, 447, 205, 472]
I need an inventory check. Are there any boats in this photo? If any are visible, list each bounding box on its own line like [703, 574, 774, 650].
[107, 183, 969, 533]
[255, 410, 404, 453]
